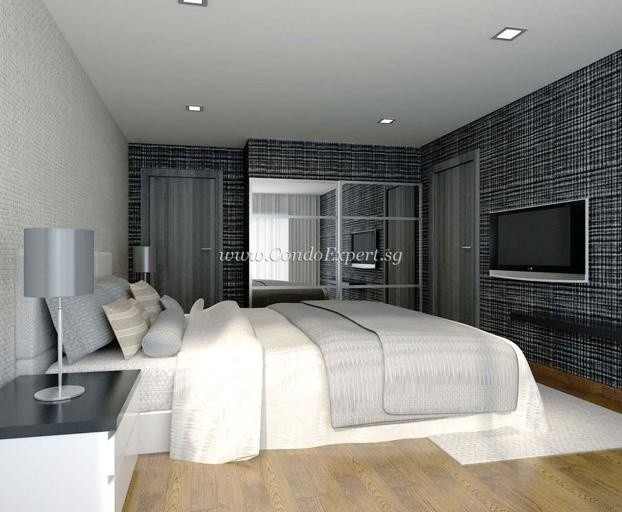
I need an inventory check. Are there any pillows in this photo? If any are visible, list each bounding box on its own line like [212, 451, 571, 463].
[45, 276, 184, 364]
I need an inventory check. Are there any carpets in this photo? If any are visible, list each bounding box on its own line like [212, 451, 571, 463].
[427, 380, 622, 463]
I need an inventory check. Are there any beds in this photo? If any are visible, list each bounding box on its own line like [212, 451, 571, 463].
[16, 245, 549, 466]
[253, 286, 330, 306]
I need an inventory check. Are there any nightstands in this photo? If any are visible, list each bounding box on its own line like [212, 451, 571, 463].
[0, 369, 151, 512]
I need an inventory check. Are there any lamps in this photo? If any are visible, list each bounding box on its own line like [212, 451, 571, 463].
[132, 244, 156, 281]
[24, 224, 94, 402]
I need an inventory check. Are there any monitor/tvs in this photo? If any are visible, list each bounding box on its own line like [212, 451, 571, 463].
[350, 228, 378, 269]
[488, 197, 590, 286]
